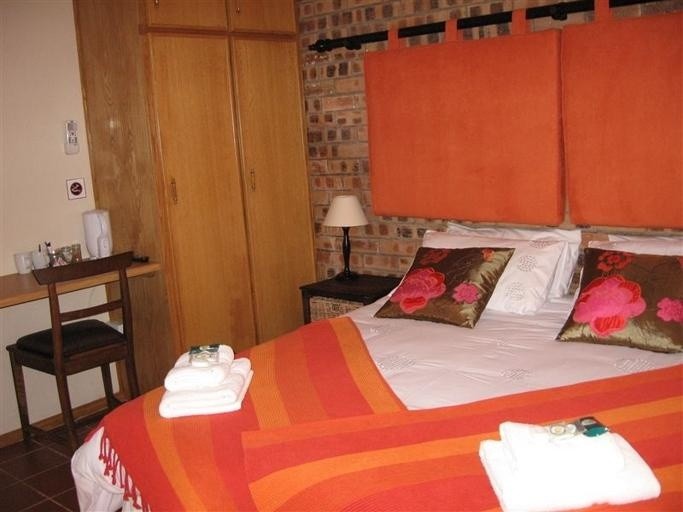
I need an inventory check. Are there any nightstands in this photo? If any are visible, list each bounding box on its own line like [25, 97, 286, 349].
[298, 273, 404, 326]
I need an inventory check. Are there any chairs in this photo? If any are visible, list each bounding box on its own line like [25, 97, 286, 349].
[6, 249, 141, 454]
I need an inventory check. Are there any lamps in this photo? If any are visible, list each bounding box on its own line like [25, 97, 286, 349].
[321, 195, 369, 282]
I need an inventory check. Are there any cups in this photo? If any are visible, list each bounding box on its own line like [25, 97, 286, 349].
[14, 253, 33, 274]
[31, 250, 50, 269]
[50, 244, 82, 268]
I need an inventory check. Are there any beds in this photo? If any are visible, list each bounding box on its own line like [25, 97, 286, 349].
[70, 224, 683, 511]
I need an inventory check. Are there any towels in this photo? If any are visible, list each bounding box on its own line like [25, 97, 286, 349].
[158, 344, 254, 419]
[479, 421, 661, 511]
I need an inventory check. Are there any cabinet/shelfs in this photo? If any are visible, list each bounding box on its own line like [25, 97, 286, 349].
[71, 0, 319, 409]
